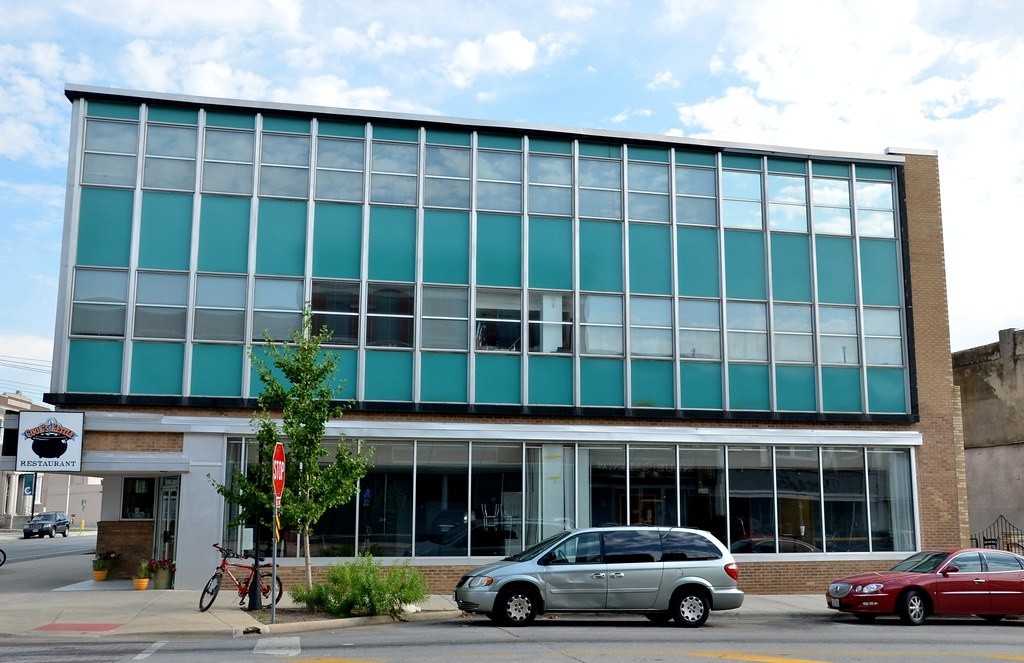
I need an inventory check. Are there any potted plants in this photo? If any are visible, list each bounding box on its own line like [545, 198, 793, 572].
[131, 559, 151, 590]
[92, 557, 110, 581]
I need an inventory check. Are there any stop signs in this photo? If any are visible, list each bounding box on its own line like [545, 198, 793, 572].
[273, 443, 286, 499]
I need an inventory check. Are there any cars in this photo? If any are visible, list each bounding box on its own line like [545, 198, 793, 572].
[726, 535, 823, 552]
[825, 548, 1024, 625]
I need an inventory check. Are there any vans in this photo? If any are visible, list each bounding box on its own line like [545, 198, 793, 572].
[453, 528, 744, 627]
[405, 517, 572, 556]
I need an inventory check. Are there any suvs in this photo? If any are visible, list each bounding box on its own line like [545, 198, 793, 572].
[23, 511, 70, 538]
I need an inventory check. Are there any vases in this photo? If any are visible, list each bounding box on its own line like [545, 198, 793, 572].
[154, 568, 173, 589]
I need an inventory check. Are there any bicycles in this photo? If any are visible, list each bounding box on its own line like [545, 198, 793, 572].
[199, 543, 283, 612]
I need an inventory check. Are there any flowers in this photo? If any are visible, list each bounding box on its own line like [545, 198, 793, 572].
[148, 560, 176, 574]
